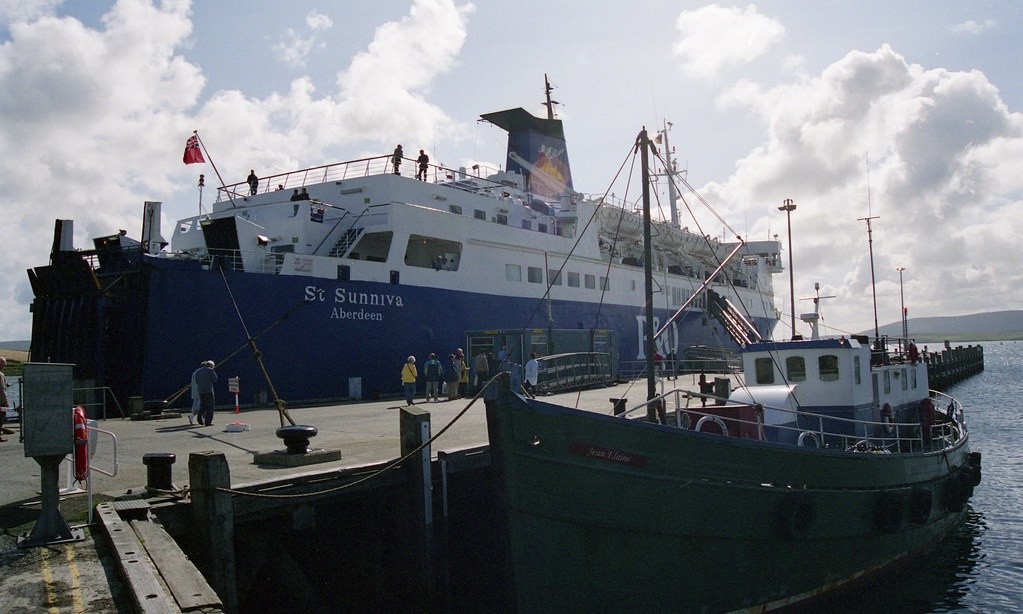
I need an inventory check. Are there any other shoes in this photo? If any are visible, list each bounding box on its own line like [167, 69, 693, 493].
[407, 402, 415, 406]
[188, 415, 194, 425]
[426, 396, 429, 401]
[532, 394, 535, 399]
[676, 378, 678, 379]
[434, 398, 438, 402]
[667, 377, 670, 381]
[0, 436, 8, 442]
[197, 418, 204, 425]
[205, 423, 214, 426]
[448, 395, 462, 401]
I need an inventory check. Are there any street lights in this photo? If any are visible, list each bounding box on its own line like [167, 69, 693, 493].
[897, 268, 906, 349]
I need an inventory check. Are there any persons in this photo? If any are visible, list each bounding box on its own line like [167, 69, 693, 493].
[401, 356, 417, 406]
[654, 348, 679, 381]
[417, 150, 429, 182]
[391, 145, 404, 174]
[424, 353, 443, 402]
[277, 185, 285, 190]
[289, 187, 310, 201]
[196, 360, 218, 427]
[444, 346, 506, 401]
[0, 356, 16, 442]
[524, 352, 538, 399]
[431, 255, 456, 271]
[247, 170, 258, 196]
[188, 361, 205, 426]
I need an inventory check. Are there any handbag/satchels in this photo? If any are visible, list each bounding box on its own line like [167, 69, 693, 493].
[441, 381, 448, 394]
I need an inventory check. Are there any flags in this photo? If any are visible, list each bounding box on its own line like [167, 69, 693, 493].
[183, 135, 205, 165]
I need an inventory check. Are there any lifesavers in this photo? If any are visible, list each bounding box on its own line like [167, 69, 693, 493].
[947, 405, 954, 417]
[875, 491, 904, 533]
[778, 491, 817, 543]
[74, 406, 87, 481]
[945, 451, 981, 512]
[883, 402, 895, 433]
[909, 343, 920, 366]
[694, 416, 729, 437]
[798, 431, 821, 448]
[911, 490, 933, 524]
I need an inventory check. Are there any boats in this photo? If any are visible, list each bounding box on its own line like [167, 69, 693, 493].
[25, 72, 785, 418]
[483, 117, 981, 614]
